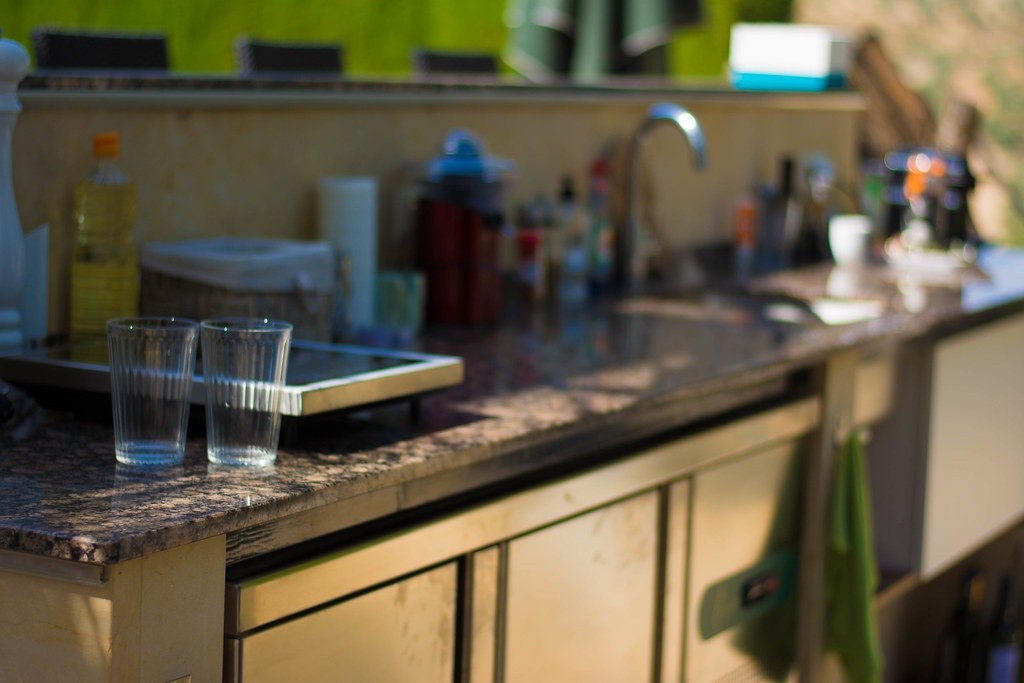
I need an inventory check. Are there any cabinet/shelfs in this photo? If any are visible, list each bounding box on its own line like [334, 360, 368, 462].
[0, 304, 1024, 682]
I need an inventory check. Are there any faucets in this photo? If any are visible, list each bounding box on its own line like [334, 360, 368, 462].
[620, 98, 709, 299]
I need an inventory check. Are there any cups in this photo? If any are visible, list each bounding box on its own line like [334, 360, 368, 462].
[200, 317, 293, 466]
[376, 270, 427, 346]
[105, 317, 200, 468]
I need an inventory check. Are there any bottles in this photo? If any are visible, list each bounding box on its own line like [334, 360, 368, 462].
[520, 159, 616, 309]
[68, 133, 141, 363]
[319, 177, 378, 344]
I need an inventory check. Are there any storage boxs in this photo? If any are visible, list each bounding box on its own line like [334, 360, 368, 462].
[728, 22, 853, 93]
